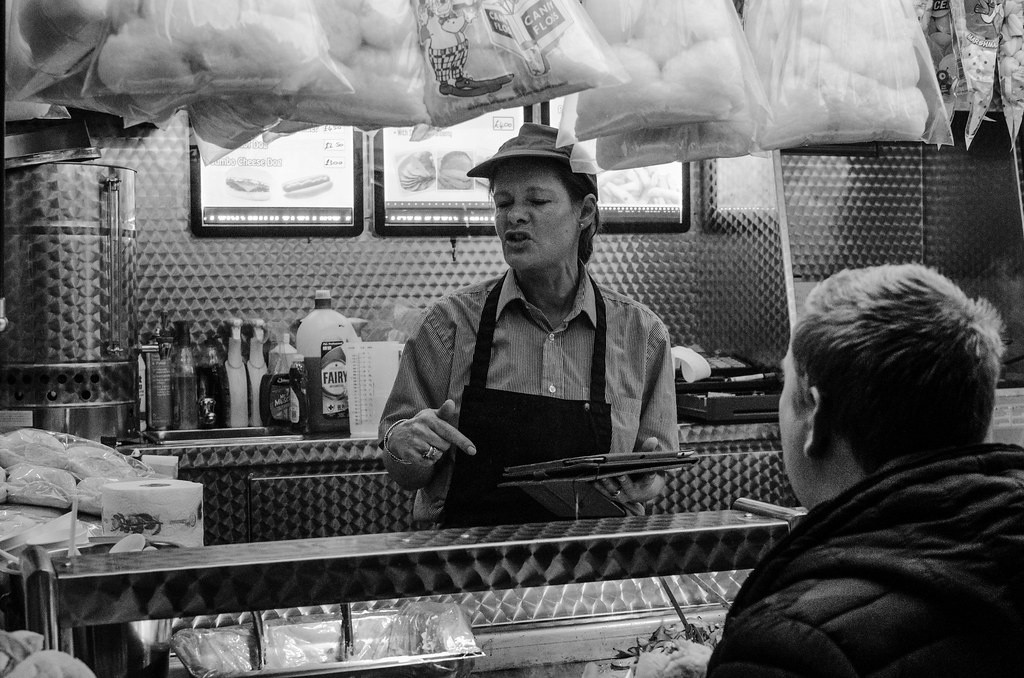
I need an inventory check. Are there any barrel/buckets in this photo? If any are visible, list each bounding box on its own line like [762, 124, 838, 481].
[5, 162, 142, 361]
[0, 540, 184, 678]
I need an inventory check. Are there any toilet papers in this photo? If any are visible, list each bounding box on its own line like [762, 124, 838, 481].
[102, 479, 204, 549]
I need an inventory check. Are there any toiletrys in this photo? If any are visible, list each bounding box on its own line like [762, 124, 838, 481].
[131, 287, 360, 440]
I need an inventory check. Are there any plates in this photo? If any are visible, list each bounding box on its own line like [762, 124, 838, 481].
[109, 534, 150, 553]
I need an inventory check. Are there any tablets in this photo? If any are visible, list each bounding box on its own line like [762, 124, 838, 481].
[505, 450, 695, 472]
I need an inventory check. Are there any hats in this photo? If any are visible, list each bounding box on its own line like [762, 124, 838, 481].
[465, 121, 598, 200]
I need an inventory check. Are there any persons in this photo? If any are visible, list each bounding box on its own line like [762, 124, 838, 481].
[706, 263, 1024, 678]
[377, 123, 679, 529]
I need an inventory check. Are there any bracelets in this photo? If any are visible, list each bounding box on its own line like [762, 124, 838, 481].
[384, 419, 412, 464]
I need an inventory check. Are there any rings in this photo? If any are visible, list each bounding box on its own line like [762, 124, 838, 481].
[423, 446, 436, 459]
[611, 488, 622, 501]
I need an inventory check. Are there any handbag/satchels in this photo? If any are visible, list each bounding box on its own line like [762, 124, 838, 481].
[408, 0, 632, 127]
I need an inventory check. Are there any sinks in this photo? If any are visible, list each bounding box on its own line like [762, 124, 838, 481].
[133, 425, 305, 446]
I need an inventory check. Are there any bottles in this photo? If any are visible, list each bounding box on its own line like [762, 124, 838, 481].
[138, 291, 360, 434]
